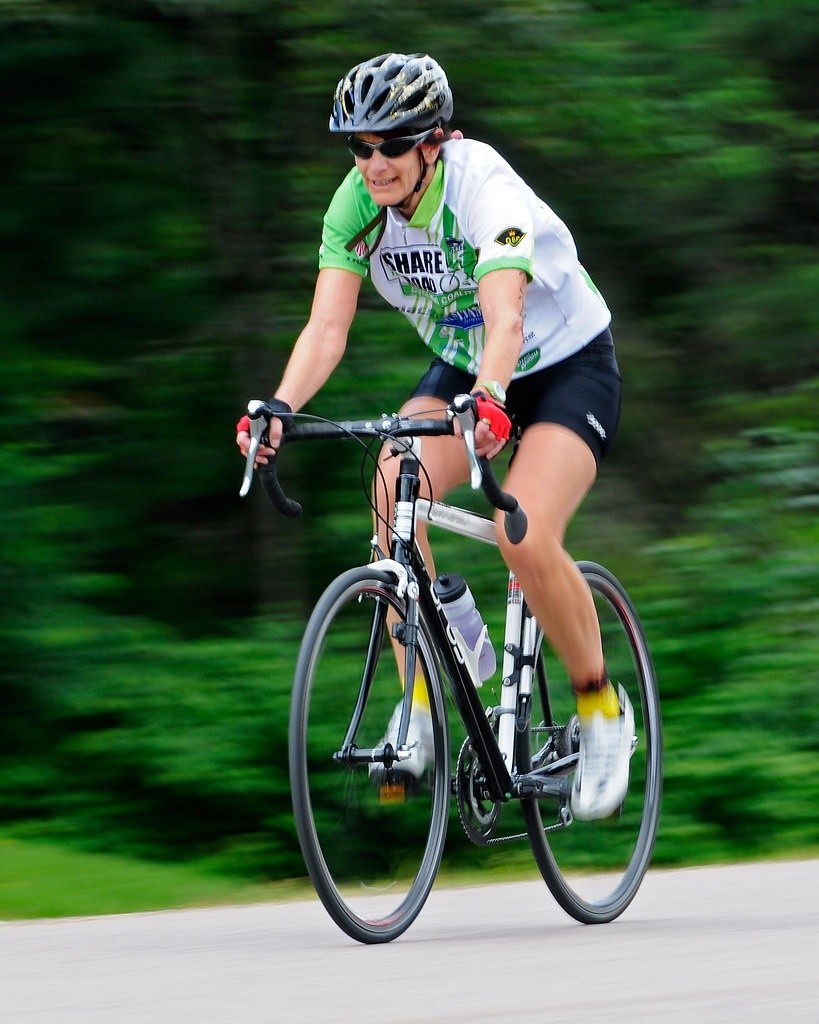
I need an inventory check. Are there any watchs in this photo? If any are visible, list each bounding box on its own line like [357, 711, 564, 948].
[473, 380, 507, 404]
[236, 54, 635, 823]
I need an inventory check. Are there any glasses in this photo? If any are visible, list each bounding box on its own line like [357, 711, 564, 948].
[346, 127, 438, 159]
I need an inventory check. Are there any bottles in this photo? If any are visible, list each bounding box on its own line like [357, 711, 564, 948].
[433, 572, 497, 682]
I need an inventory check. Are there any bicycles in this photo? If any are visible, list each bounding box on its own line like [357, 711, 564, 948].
[237, 392, 664, 946]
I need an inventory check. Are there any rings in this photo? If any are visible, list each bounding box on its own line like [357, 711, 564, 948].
[481, 418, 490, 424]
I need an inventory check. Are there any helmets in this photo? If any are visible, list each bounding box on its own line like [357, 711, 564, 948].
[329, 53, 453, 132]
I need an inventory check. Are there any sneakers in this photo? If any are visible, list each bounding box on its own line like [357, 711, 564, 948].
[571, 678, 634, 820]
[367, 698, 435, 786]
